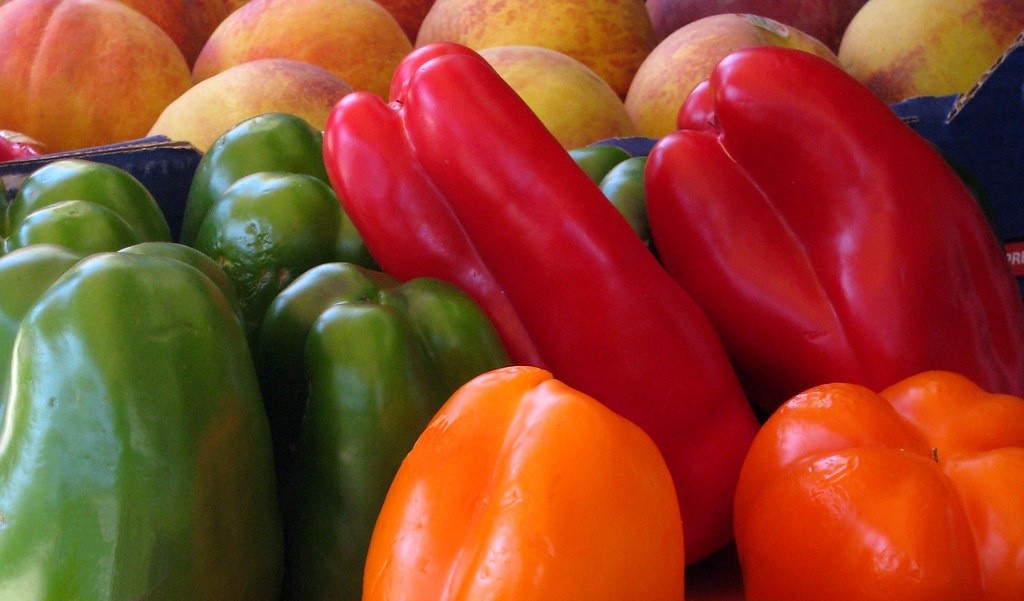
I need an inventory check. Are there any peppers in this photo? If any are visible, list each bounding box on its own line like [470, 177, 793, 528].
[1, 44, 1024, 601]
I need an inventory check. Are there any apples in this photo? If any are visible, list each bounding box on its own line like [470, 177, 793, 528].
[0, 0, 1024, 154]
